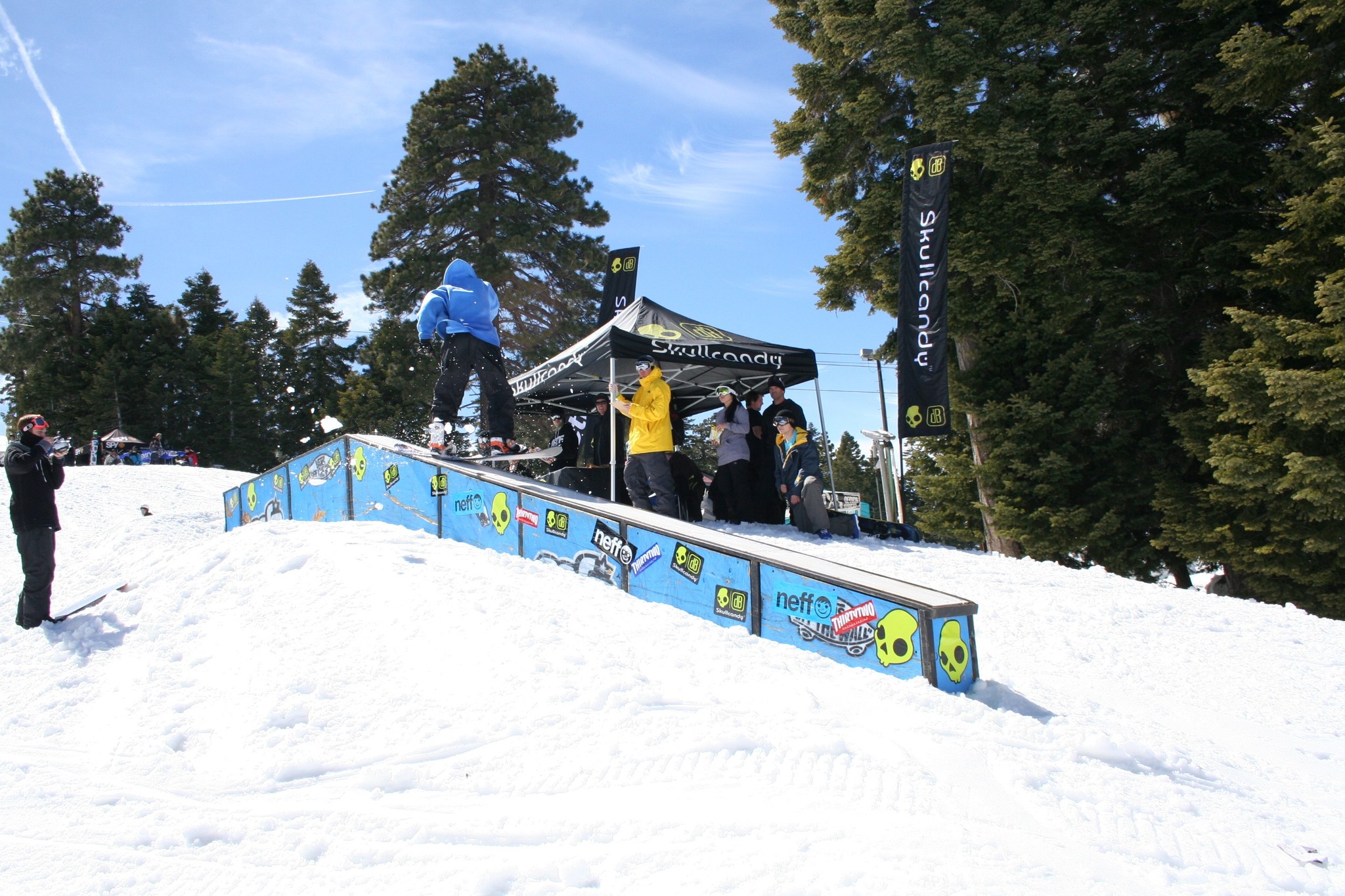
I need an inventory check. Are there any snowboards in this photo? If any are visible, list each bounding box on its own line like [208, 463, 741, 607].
[393, 440, 564, 462]
[50, 577, 128, 621]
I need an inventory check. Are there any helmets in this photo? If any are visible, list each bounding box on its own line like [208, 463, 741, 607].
[637, 355, 655, 370]
[550, 406, 565, 423]
[719, 382, 735, 396]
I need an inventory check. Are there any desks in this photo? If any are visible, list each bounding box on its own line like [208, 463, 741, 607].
[534, 466, 633, 509]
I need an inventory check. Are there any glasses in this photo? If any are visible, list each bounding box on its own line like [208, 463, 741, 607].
[715, 386, 736, 397]
[595, 400, 608, 406]
[773, 417, 795, 426]
[17, 414, 49, 437]
[550, 414, 561, 420]
[634, 361, 655, 372]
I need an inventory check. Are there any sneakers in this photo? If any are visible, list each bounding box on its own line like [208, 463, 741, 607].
[819, 527, 832, 540]
[490, 436, 528, 457]
[428, 422, 453, 456]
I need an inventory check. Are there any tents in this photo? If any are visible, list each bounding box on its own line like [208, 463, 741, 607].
[74, 429, 147, 466]
[506, 295, 819, 420]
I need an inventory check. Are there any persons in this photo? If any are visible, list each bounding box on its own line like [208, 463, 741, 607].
[502, 460, 534, 479]
[665, 449, 706, 523]
[89, 430, 103, 466]
[155, 433, 164, 465]
[4, 414, 68, 629]
[743, 390, 765, 524]
[104, 449, 120, 466]
[8, 434, 16, 445]
[711, 382, 751, 524]
[149, 436, 159, 465]
[583, 392, 624, 468]
[183, 446, 198, 467]
[608, 354, 675, 517]
[16, 432, 21, 442]
[124, 445, 143, 466]
[762, 375, 807, 526]
[417, 258, 528, 458]
[56, 431, 65, 466]
[470, 430, 504, 469]
[772, 409, 833, 540]
[528, 407, 578, 473]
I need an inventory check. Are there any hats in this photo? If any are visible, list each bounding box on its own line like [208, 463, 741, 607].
[595, 393, 609, 408]
[775, 410, 795, 427]
[768, 376, 785, 392]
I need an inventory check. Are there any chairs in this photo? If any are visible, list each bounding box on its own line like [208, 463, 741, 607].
[674, 492, 690, 523]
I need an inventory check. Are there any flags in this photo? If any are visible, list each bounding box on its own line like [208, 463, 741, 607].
[597, 246, 641, 329]
[898, 140, 952, 436]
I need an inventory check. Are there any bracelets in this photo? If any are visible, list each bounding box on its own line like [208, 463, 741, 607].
[615, 392, 620, 398]
